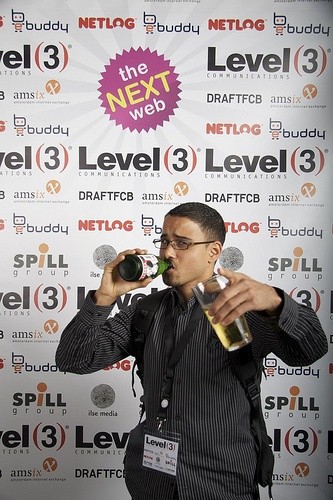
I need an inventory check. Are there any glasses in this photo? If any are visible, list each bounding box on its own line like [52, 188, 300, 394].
[153, 238, 216, 250]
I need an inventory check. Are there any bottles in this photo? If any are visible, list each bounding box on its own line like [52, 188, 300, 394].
[117, 254, 173, 282]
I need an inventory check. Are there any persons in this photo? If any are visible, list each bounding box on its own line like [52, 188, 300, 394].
[54, 203, 329, 500]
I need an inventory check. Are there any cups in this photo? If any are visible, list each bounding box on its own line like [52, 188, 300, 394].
[191, 274, 253, 352]
